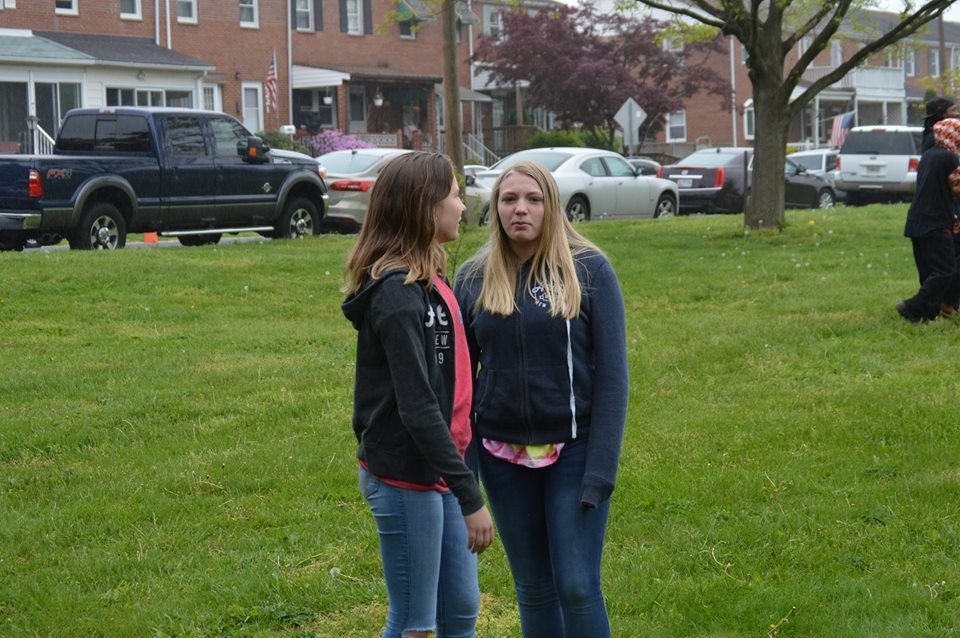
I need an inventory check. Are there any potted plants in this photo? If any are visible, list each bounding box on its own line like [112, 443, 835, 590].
[372, 92, 385, 107]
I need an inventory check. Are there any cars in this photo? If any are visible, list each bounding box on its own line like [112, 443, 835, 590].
[834, 124, 926, 206]
[785, 150, 848, 202]
[470, 148, 680, 224]
[657, 147, 835, 216]
[316, 147, 492, 234]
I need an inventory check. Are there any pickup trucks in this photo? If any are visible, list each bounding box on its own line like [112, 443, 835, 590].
[0, 106, 329, 252]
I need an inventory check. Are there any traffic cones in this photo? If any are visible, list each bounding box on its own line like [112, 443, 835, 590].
[143, 232, 159, 244]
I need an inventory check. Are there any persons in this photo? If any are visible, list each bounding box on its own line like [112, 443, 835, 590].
[896, 96, 959, 321]
[452, 161, 629, 638]
[341, 152, 494, 638]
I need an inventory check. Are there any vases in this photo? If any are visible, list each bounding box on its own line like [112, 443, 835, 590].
[323, 96, 333, 105]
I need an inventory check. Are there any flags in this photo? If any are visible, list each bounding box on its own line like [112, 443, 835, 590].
[831, 111, 856, 148]
[266, 53, 277, 112]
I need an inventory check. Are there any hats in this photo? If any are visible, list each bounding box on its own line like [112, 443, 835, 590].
[921, 98, 954, 120]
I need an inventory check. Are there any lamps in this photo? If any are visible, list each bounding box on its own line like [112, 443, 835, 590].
[236, 101, 241, 113]
[26, 115, 40, 131]
[236, 72, 240, 79]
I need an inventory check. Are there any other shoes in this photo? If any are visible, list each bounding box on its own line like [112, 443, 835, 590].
[896, 302, 919, 323]
[940, 303, 956, 317]
